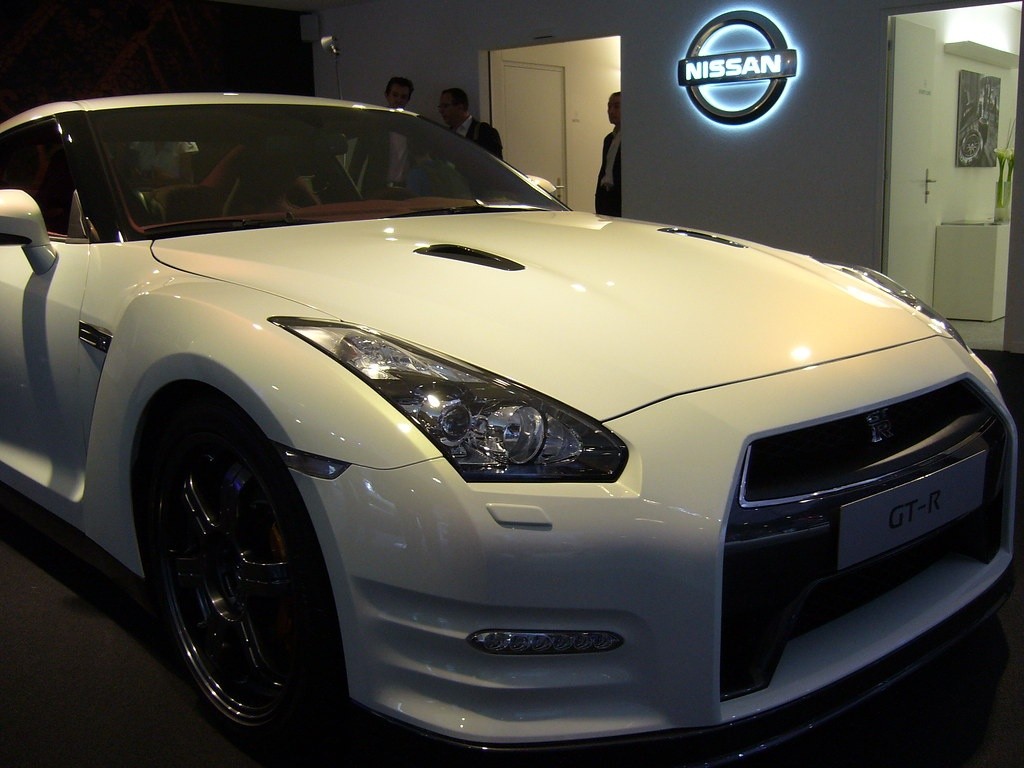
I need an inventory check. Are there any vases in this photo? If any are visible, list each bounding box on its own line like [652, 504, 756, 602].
[994, 178, 1013, 224]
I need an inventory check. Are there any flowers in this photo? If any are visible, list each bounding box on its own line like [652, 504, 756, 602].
[994, 117, 1018, 210]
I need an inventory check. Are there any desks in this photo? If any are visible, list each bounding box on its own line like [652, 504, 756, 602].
[931, 223, 1011, 320]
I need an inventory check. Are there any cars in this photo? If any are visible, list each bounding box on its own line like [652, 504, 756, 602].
[1, 91, 1021, 768]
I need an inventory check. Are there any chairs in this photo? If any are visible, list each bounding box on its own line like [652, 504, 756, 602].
[146, 182, 228, 227]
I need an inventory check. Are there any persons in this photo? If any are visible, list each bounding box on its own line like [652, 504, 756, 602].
[367, 76, 431, 198]
[437, 86, 506, 198]
[592, 90, 623, 219]
[0, 61, 259, 224]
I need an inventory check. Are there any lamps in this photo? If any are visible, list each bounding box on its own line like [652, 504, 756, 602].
[321, 35, 338, 55]
